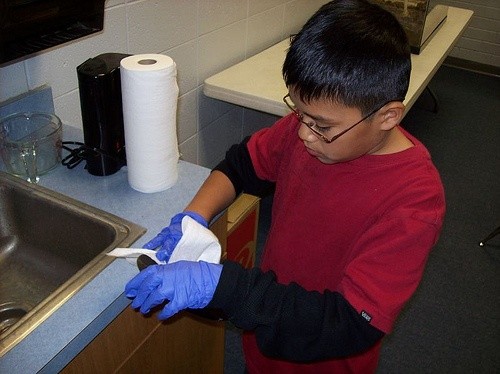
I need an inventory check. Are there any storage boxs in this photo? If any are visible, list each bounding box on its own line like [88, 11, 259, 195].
[228, 193, 261, 269]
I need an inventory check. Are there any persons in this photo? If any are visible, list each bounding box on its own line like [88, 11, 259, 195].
[125, 0, 446, 374]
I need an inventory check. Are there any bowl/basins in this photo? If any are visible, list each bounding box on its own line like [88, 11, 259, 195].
[0, 112, 62, 183]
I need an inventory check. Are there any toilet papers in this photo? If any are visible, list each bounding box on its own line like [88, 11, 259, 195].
[120, 53, 180, 194]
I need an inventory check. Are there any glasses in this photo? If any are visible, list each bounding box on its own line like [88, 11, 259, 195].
[281, 91, 393, 145]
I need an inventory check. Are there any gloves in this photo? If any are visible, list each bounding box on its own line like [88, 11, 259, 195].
[125, 265, 225, 322]
[140, 210, 209, 259]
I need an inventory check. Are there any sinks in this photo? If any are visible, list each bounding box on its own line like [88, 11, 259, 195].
[0, 171, 147, 357]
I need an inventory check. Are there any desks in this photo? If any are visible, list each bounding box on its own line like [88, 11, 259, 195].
[203, 6, 474, 127]
